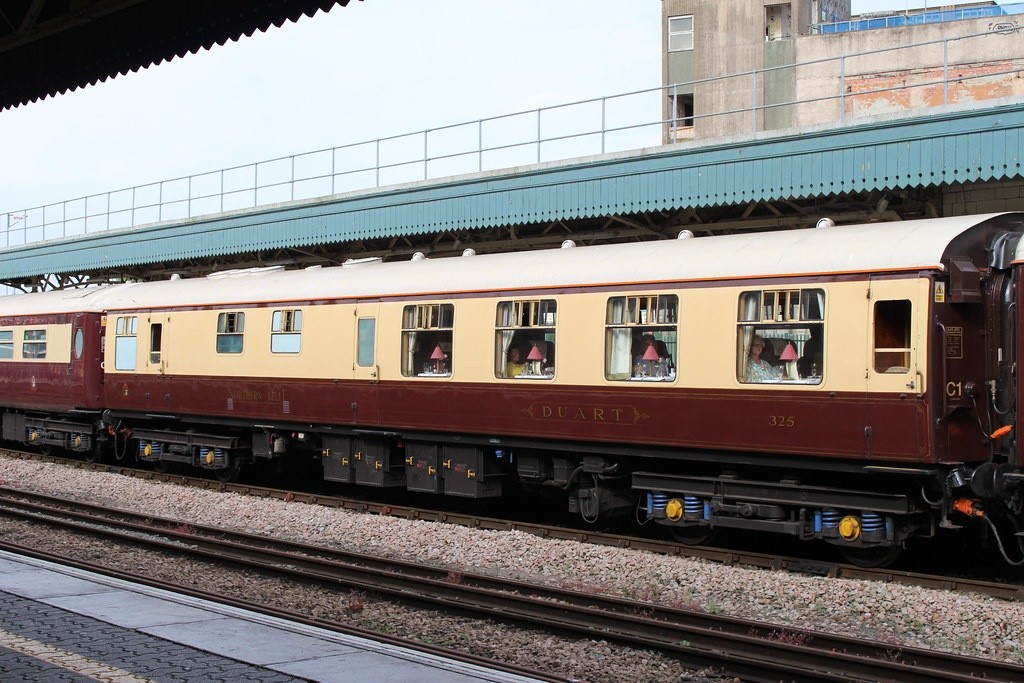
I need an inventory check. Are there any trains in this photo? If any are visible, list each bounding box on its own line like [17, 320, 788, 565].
[0, 210, 1024, 577]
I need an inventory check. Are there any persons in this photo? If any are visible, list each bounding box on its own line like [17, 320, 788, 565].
[506, 345, 526, 377]
[746, 334, 779, 383]
[632, 334, 668, 377]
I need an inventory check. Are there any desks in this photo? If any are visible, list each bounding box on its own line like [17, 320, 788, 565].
[514, 373, 554, 379]
[761, 379, 821, 383]
[418, 373, 451, 378]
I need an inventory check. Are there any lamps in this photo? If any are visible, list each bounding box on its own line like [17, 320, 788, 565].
[779, 341, 798, 381]
[630, 376, 673, 382]
[430, 346, 444, 373]
[643, 343, 660, 377]
[527, 343, 544, 375]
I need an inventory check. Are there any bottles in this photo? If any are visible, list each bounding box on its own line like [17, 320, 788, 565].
[773, 363, 784, 380]
[635, 363, 647, 378]
[433, 365, 436, 373]
[810, 360, 818, 376]
[655, 353, 674, 377]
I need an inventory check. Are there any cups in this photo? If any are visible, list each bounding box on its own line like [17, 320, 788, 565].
[423, 362, 430, 374]
[521, 363, 532, 376]
[668, 373, 676, 377]
